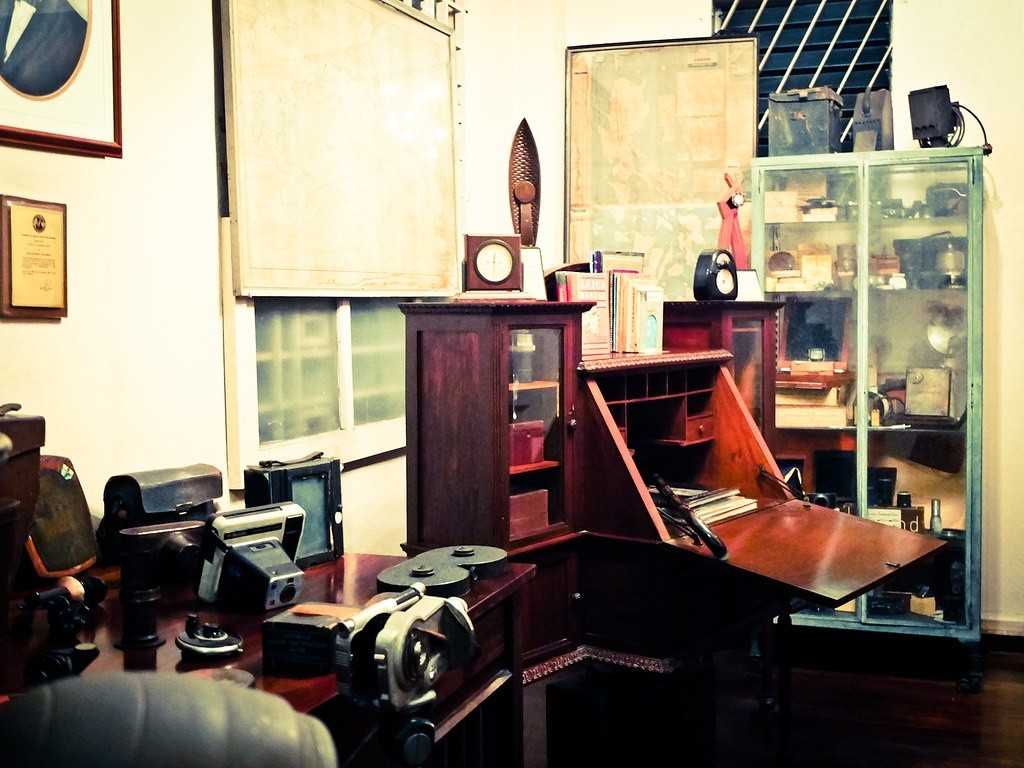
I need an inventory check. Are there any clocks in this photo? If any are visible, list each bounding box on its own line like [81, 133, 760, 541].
[462, 233, 524, 292]
[693, 249, 738, 300]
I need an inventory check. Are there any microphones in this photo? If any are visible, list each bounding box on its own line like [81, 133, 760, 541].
[20, 575, 108, 611]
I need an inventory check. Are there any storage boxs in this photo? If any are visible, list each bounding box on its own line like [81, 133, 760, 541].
[245, 450, 345, 571]
[509, 417, 544, 467]
[510, 488, 548, 538]
[768, 86, 843, 157]
[262, 601, 362, 677]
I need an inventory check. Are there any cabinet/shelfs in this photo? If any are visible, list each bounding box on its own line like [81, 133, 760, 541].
[1, 553, 537, 767]
[750, 145, 982, 642]
[396, 302, 585, 684]
[662, 301, 784, 461]
[575, 350, 950, 676]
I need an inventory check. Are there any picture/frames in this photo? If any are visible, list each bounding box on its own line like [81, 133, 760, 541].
[0, 0, 122, 158]
